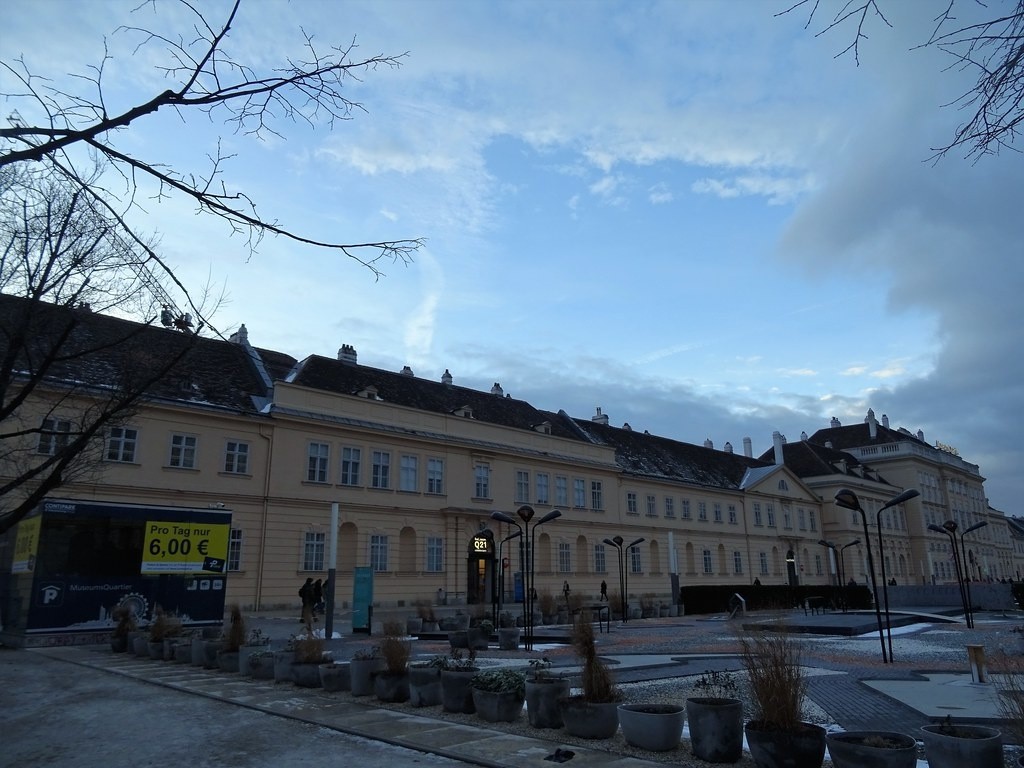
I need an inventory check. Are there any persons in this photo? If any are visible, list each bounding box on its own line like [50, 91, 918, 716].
[962, 575, 1014, 584]
[298, 577, 329, 623]
[563, 580, 572, 604]
[600, 581, 609, 602]
[847, 577, 898, 586]
[754, 576, 761, 586]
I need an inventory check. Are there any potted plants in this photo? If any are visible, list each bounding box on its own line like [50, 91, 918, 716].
[111, 587, 1003, 768]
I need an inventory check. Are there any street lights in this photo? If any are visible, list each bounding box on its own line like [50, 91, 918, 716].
[833, 487, 922, 664]
[817, 538, 862, 613]
[926, 520, 989, 629]
[602, 535, 646, 624]
[489, 504, 562, 652]
[479, 526, 525, 632]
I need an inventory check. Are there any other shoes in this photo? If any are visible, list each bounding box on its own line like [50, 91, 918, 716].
[314, 618, 317, 621]
[300, 619, 305, 622]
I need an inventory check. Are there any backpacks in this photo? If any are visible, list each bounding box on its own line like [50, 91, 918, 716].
[299, 589, 304, 597]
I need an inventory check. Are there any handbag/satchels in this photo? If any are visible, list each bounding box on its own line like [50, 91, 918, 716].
[314, 602, 326, 611]
[567, 590, 571, 593]
